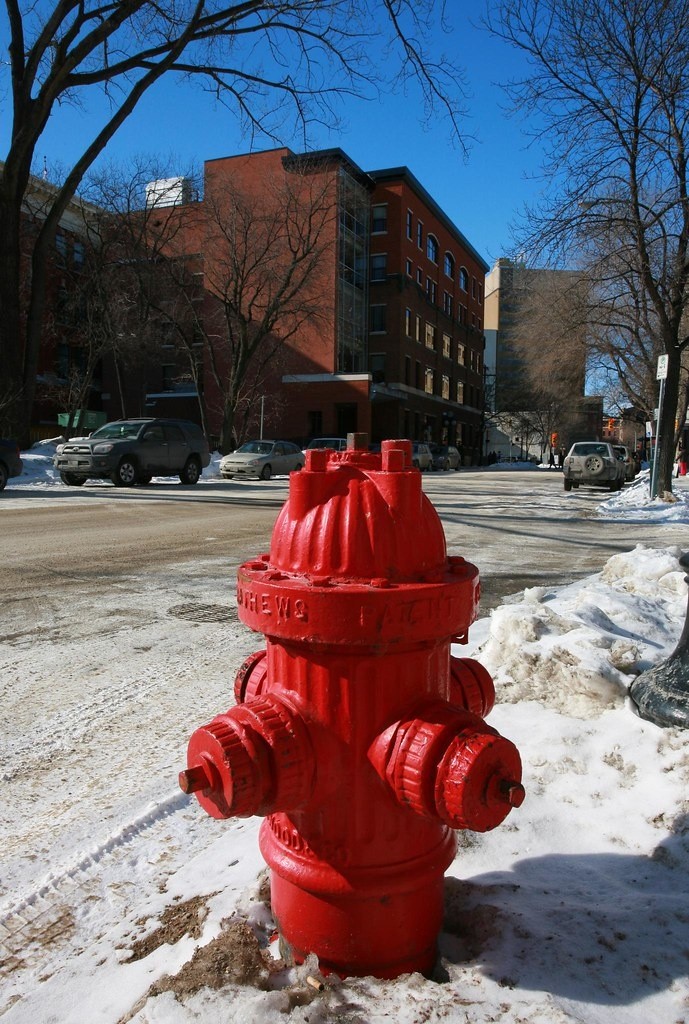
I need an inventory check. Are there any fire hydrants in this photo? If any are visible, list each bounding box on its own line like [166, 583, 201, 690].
[179, 431, 535, 985]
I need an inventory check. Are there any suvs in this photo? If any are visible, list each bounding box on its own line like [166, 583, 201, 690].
[53, 416, 212, 488]
[563, 441, 641, 492]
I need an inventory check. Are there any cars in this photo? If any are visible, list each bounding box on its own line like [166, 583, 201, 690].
[408, 442, 434, 473]
[219, 439, 306, 482]
[430, 445, 462, 472]
[0, 439, 23, 490]
[301, 438, 347, 460]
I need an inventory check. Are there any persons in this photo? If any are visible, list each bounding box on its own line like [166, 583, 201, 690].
[675, 447, 687, 478]
[548, 453, 557, 469]
[478, 448, 501, 467]
[558, 453, 565, 469]
